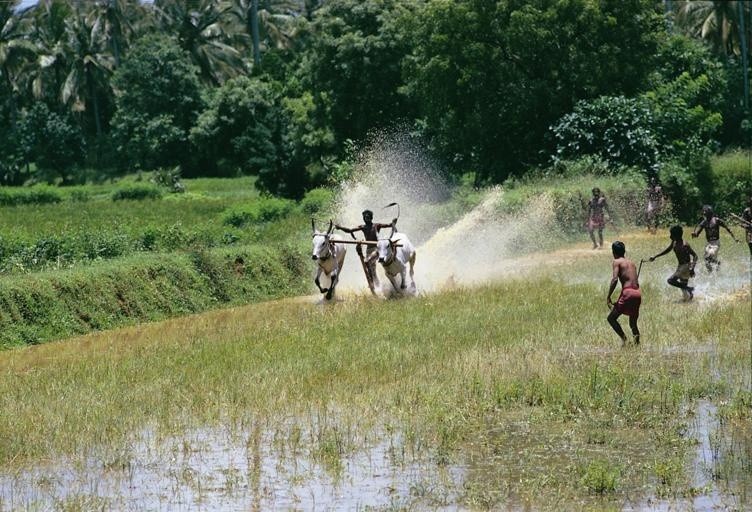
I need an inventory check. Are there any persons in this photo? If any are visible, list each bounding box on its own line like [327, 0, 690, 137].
[691, 205, 738, 271]
[606, 240, 641, 347]
[579, 188, 612, 249]
[335, 209, 397, 297]
[649, 225, 698, 299]
[740, 196, 751, 257]
[644, 177, 663, 233]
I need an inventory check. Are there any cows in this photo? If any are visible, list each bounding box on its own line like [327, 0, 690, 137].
[307, 218, 357, 302]
[374, 202, 417, 296]
